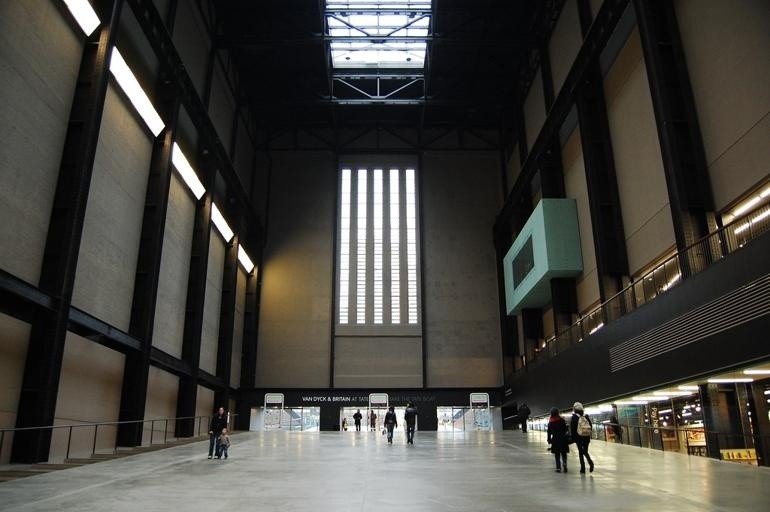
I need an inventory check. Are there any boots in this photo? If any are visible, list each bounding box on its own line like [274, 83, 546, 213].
[580, 460, 594, 474]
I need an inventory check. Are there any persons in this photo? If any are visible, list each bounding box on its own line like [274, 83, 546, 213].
[217, 428, 230, 459]
[353, 409, 362, 431]
[519, 402, 531, 433]
[569, 402, 594, 473]
[405, 401, 419, 444]
[207, 407, 227, 459]
[368, 410, 376, 431]
[342, 417, 348, 431]
[547, 407, 569, 472]
[384, 405, 397, 443]
[611, 417, 620, 440]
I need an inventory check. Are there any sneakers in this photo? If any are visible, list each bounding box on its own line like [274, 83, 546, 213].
[208, 456, 211, 459]
[214, 456, 219, 459]
[555, 465, 567, 473]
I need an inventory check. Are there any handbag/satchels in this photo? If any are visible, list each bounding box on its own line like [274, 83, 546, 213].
[563, 429, 573, 445]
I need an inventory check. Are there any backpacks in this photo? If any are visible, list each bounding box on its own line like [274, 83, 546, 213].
[575, 413, 591, 437]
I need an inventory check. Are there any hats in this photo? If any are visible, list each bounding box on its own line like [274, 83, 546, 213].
[574, 402, 583, 410]
